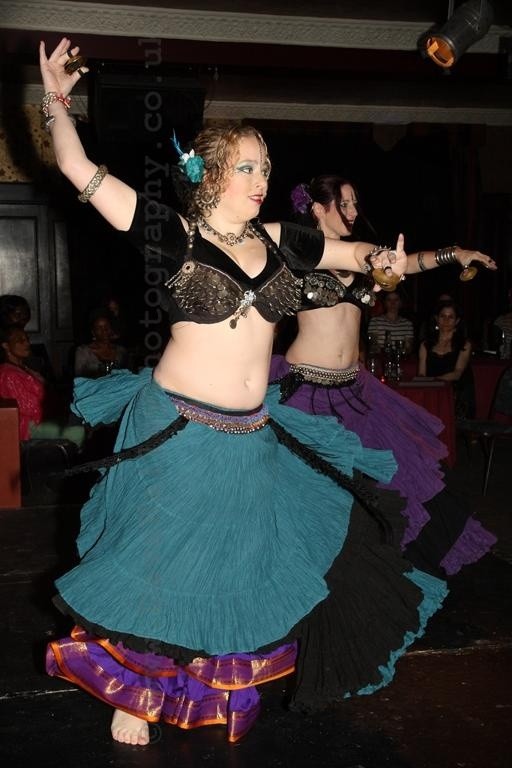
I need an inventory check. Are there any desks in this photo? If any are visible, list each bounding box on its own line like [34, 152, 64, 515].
[367, 344, 460, 474]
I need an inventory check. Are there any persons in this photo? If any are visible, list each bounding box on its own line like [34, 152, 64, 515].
[1, 283, 511, 515]
[267, 172, 498, 585]
[36, 35, 408, 750]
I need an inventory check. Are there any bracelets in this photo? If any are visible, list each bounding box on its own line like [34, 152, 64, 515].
[40, 92, 71, 117]
[40, 113, 77, 136]
[434, 246, 458, 266]
[417, 250, 428, 273]
[76, 164, 109, 204]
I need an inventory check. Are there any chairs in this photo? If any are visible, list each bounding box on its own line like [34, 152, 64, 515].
[1, 398, 102, 630]
[454, 367, 512, 507]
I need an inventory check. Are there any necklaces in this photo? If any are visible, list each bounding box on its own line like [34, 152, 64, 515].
[195, 217, 257, 247]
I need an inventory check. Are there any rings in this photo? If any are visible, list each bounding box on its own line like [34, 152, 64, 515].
[75, 65, 84, 77]
[65, 49, 81, 62]
[487, 256, 492, 261]
[463, 265, 472, 272]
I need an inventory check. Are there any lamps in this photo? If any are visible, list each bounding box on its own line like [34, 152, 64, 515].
[415, 1, 491, 69]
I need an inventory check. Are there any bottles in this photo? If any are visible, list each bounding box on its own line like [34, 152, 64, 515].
[364, 330, 407, 378]
